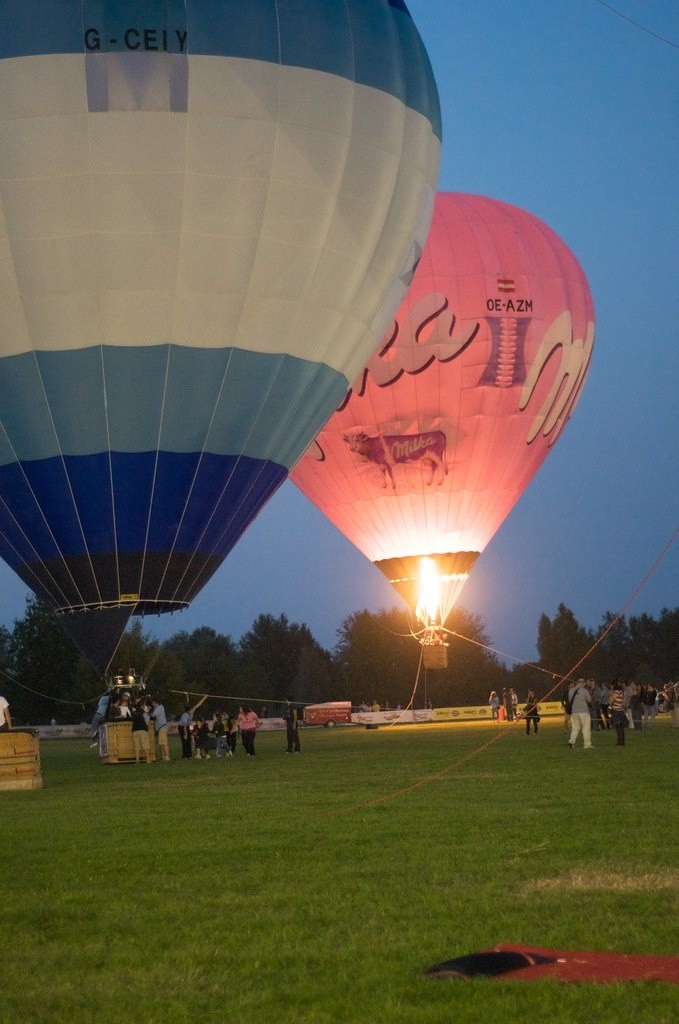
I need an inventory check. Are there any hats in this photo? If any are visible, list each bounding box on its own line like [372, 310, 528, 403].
[123, 691, 131, 698]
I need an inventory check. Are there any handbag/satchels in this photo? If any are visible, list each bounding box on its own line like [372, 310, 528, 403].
[497, 706, 501, 709]
[154, 731, 159, 736]
[565, 703, 572, 715]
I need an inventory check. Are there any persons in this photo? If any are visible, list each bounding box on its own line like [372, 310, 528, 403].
[525, 688, 538, 735]
[489, 687, 518, 721]
[284, 697, 301, 754]
[0, 696, 12, 732]
[420, 634, 449, 646]
[357, 699, 432, 713]
[561, 678, 679, 749]
[91, 686, 269, 763]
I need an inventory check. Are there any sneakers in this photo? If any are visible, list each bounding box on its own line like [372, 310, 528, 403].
[567, 739, 576, 748]
[582, 743, 598, 750]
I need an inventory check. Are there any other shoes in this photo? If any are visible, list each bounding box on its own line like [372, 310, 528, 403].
[294, 750, 302, 754]
[247, 753, 255, 758]
[89, 742, 99, 748]
[135, 762, 140, 766]
[285, 748, 292, 753]
[161, 756, 171, 761]
[225, 750, 233, 758]
[615, 743, 624, 747]
[147, 762, 154, 765]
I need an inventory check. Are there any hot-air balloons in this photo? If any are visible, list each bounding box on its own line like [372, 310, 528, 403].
[286, 192, 597, 675]
[1, 0, 444, 765]
